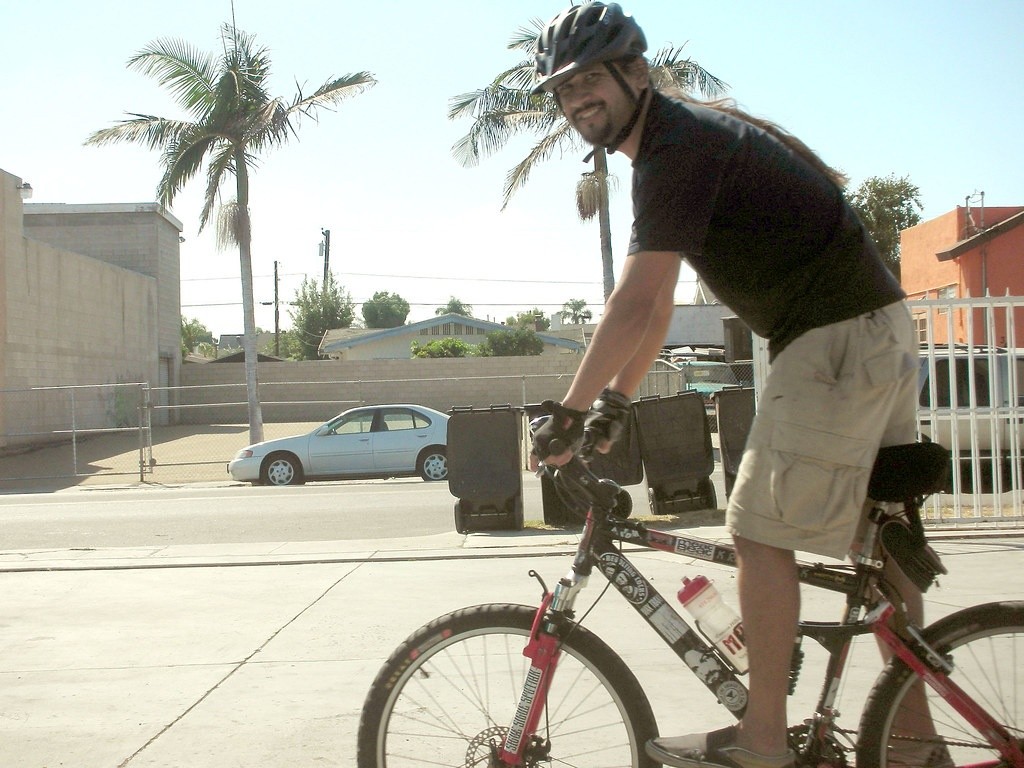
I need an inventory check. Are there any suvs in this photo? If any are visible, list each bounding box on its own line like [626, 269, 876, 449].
[907, 344, 1024, 460]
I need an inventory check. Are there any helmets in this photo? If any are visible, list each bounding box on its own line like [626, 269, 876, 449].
[530, 1, 647, 95]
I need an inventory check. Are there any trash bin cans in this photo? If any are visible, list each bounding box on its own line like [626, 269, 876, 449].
[522, 403, 632, 526]
[447, 403, 527, 533]
[710, 384, 757, 503]
[632, 388, 719, 515]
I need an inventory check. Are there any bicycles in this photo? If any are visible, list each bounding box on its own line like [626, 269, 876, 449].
[354, 410, 1024, 768]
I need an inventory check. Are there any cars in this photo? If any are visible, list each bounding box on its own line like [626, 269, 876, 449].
[672, 361, 748, 414]
[229, 403, 451, 487]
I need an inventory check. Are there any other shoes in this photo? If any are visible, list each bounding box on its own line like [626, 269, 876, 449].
[846, 735, 955, 768]
[645, 725, 795, 768]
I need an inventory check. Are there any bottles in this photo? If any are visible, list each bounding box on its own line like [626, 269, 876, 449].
[677, 574, 749, 674]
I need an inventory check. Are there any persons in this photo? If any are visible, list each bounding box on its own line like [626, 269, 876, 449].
[530, 1, 955, 768]
